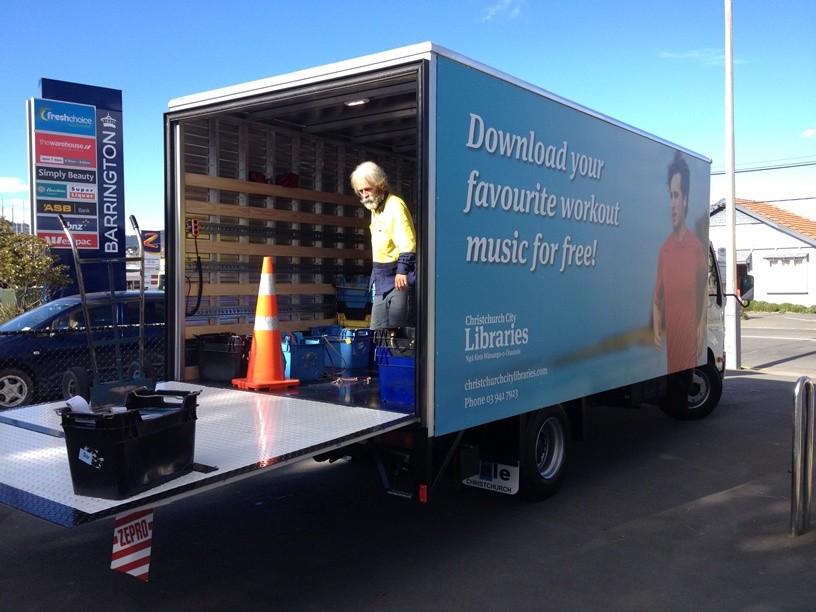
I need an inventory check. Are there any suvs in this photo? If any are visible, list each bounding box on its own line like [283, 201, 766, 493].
[0, 289, 166, 409]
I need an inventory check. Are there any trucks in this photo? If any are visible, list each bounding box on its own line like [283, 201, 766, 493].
[0, 42, 756, 537]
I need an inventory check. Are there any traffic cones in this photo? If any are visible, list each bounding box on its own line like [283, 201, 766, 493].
[231, 257, 303, 390]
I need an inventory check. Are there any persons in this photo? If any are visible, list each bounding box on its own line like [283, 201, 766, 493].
[651, 156, 708, 374]
[350, 161, 416, 329]
